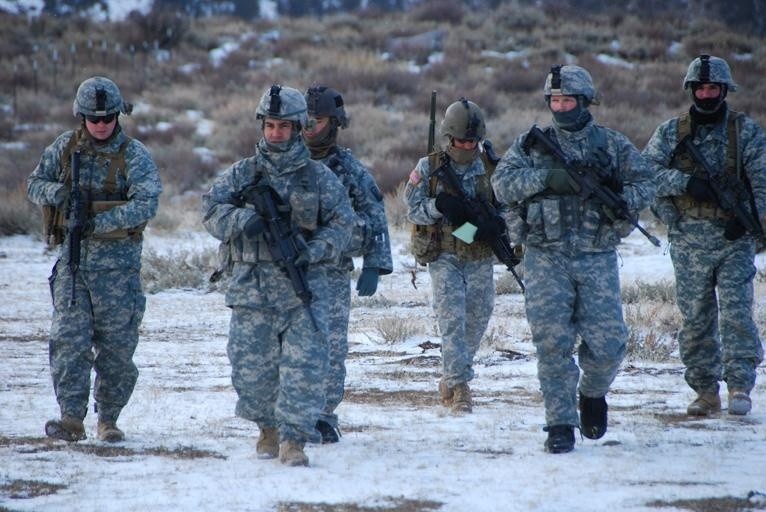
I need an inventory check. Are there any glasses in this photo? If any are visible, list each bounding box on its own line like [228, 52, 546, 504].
[84, 115, 116, 124]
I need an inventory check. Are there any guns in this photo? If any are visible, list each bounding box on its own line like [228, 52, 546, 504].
[428, 154, 526, 294]
[231, 172, 320, 335]
[520, 124, 661, 247]
[67, 149, 89, 306]
[673, 133, 761, 253]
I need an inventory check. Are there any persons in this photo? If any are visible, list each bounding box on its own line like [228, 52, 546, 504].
[26, 78, 162, 442]
[641, 54, 762, 415]
[200, 87, 355, 468]
[403, 98, 522, 417]
[293, 86, 393, 444]
[490, 65, 652, 453]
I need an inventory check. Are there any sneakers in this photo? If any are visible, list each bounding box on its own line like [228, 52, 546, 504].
[543, 424, 575, 454]
[452, 382, 472, 413]
[315, 416, 338, 443]
[438, 377, 452, 407]
[45, 410, 87, 442]
[279, 437, 309, 468]
[254, 426, 279, 459]
[727, 389, 752, 417]
[579, 386, 608, 440]
[97, 418, 125, 443]
[689, 389, 723, 414]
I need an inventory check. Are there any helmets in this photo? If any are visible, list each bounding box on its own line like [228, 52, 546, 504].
[72, 77, 126, 118]
[683, 55, 738, 92]
[254, 86, 308, 132]
[543, 64, 596, 106]
[303, 86, 350, 130]
[439, 97, 486, 141]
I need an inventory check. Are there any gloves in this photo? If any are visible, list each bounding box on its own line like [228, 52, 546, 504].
[601, 199, 617, 222]
[435, 193, 465, 230]
[482, 215, 506, 234]
[64, 218, 95, 241]
[54, 187, 70, 219]
[687, 175, 718, 206]
[545, 168, 580, 197]
[279, 249, 309, 280]
[724, 218, 747, 240]
[243, 215, 268, 244]
[356, 268, 379, 296]
